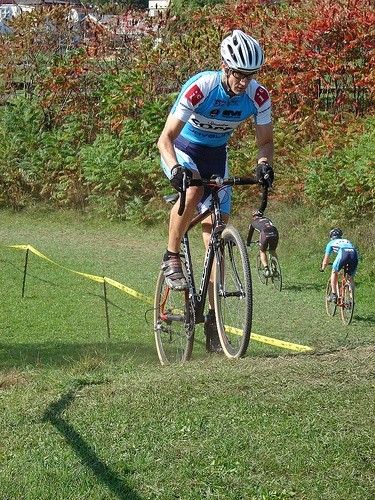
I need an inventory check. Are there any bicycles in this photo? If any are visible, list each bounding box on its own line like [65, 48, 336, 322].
[321, 260, 354, 326]
[245, 240, 282, 292]
[145, 171, 270, 365]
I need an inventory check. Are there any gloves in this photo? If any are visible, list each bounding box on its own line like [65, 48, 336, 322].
[319, 267, 324, 272]
[255, 160, 275, 187]
[246, 242, 250, 246]
[169, 163, 193, 192]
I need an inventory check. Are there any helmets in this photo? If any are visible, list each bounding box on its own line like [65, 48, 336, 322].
[221, 29, 264, 72]
[330, 228, 343, 238]
[252, 210, 263, 218]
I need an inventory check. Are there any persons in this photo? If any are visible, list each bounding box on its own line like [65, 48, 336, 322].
[322, 227, 358, 310]
[246, 211, 279, 277]
[158, 29, 274, 354]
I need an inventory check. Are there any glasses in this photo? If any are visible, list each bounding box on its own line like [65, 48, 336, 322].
[228, 68, 258, 80]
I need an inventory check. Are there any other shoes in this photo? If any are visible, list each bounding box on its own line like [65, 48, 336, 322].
[271, 268, 277, 277]
[332, 295, 337, 303]
[264, 267, 270, 278]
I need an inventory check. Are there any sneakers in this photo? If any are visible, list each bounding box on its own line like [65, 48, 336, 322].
[205, 310, 231, 353]
[160, 251, 190, 291]
